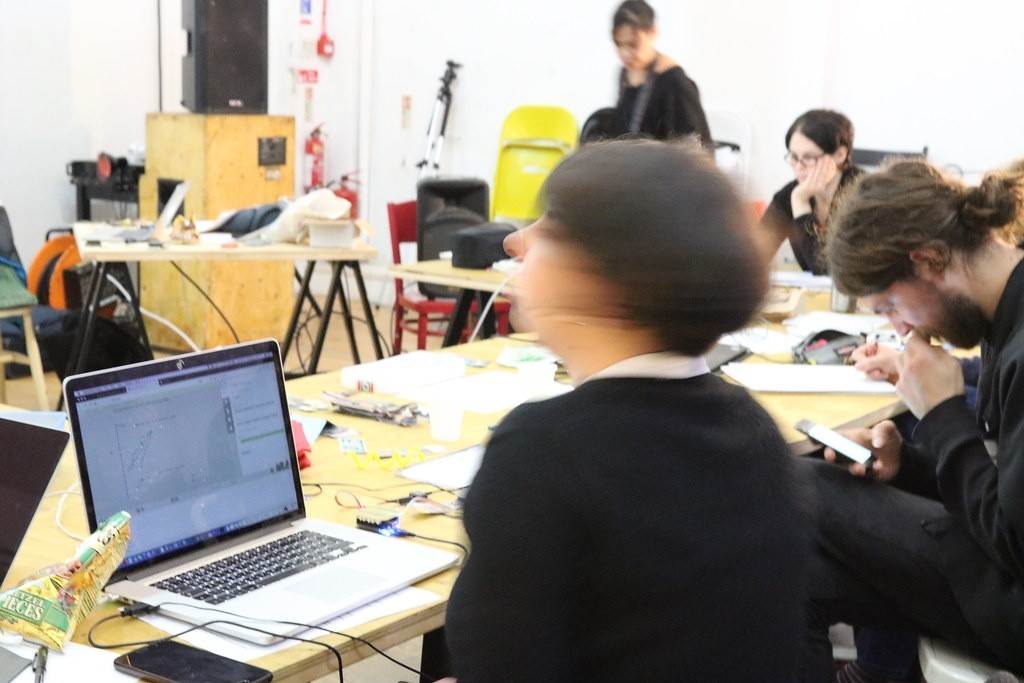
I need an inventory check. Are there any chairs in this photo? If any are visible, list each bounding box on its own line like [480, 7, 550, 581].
[0, 205, 51, 415]
[387, 202, 511, 355]
[848, 145, 932, 168]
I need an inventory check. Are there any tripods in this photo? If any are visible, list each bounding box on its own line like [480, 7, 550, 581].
[416, 59, 462, 179]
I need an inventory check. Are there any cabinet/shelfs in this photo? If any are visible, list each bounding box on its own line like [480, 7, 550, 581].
[142, 110, 296, 356]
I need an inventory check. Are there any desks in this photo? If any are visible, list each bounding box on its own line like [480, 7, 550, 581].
[57, 219, 384, 409]
[0, 334, 984, 683]
[389, 258, 829, 349]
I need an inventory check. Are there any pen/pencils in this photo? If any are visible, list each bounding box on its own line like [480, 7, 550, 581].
[31, 647, 48, 683]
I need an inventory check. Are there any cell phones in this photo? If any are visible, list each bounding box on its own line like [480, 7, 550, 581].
[794, 419, 876, 464]
[114, 638, 274, 683]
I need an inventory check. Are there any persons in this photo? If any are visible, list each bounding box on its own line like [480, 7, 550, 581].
[761, 109, 868, 278]
[611, 0, 716, 160]
[791, 159, 1024, 683]
[442, 139, 819, 683]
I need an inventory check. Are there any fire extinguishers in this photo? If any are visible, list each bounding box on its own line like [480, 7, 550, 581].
[325, 170, 363, 219]
[303, 122, 328, 193]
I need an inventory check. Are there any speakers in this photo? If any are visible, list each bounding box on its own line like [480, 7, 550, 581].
[180, 0, 268, 113]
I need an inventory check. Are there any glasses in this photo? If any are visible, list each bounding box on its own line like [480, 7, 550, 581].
[784, 151, 826, 168]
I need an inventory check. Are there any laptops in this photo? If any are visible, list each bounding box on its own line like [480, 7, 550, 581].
[63, 336, 458, 646]
[87, 181, 193, 244]
